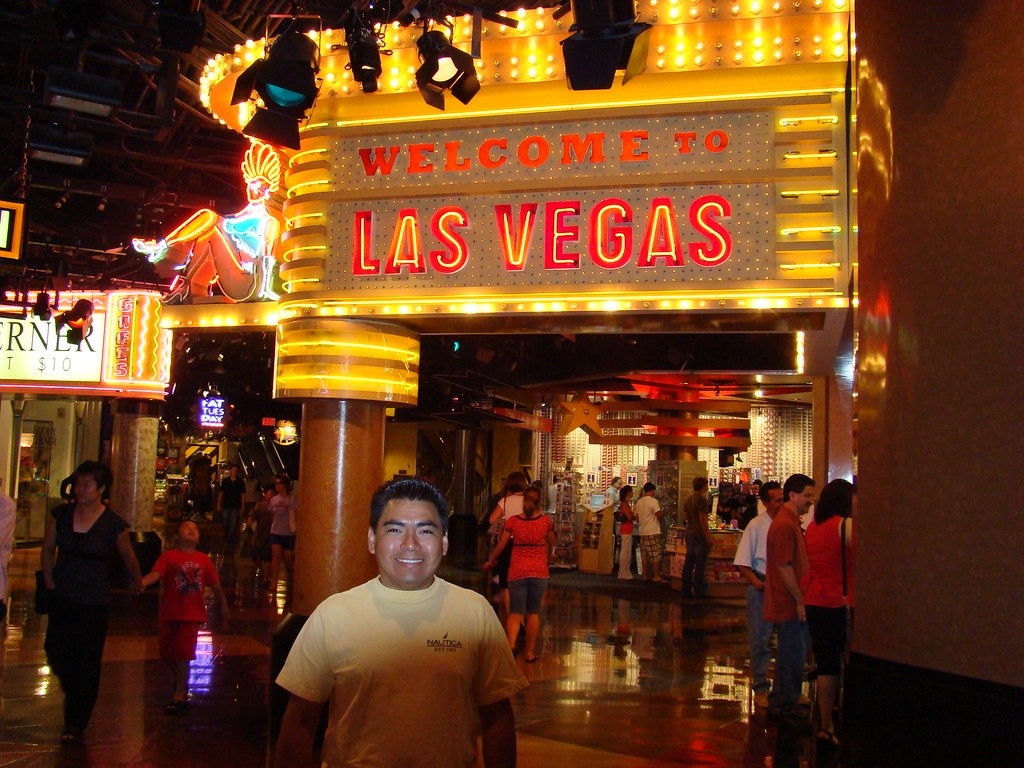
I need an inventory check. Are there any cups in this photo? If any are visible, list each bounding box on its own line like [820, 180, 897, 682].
[720, 520, 726, 529]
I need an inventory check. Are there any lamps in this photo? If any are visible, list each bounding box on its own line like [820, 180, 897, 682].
[229, 15, 322, 151]
[98, 197, 107, 210]
[53, 192, 67, 209]
[0, 270, 94, 345]
[344, 12, 382, 93]
[553, 0, 653, 91]
[416, 18, 481, 111]
[26, 1, 206, 169]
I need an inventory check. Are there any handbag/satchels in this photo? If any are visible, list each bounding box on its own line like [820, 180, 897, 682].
[289, 497, 298, 533]
[613, 511, 637, 523]
[489, 495, 508, 536]
[34, 568, 50, 615]
[844, 604, 855, 640]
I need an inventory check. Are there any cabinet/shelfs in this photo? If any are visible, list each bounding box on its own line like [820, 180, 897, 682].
[578, 504, 613, 575]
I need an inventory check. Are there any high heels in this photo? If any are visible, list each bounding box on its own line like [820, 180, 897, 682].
[817, 727, 839, 749]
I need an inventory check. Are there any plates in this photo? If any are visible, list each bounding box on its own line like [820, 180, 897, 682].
[727, 529, 739, 530]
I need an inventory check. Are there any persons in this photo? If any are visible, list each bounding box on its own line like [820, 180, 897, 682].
[193, 462, 297, 590]
[481, 470, 560, 661]
[763, 473, 815, 726]
[0, 477, 18, 620]
[634, 482, 670, 583]
[803, 478, 854, 750]
[139, 520, 232, 712]
[733, 480, 814, 708]
[717, 479, 768, 530]
[606, 477, 623, 504]
[269, 478, 532, 768]
[618, 485, 635, 580]
[42, 460, 144, 744]
[680, 477, 716, 599]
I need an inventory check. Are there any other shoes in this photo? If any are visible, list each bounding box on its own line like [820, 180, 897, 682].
[166, 695, 188, 713]
[268, 583, 279, 591]
[765, 704, 808, 725]
[682, 580, 709, 598]
[752, 685, 771, 710]
[797, 693, 811, 705]
[653, 577, 668, 584]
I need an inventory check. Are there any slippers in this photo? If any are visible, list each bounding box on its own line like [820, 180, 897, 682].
[525, 654, 537, 662]
[62, 725, 80, 740]
[511, 647, 518, 654]
